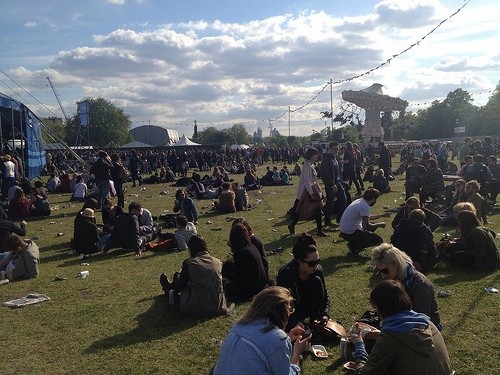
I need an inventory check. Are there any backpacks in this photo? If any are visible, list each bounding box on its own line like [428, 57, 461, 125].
[114, 162, 129, 183]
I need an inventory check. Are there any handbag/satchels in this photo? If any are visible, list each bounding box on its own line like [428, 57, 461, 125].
[357, 310, 383, 330]
[419, 241, 440, 271]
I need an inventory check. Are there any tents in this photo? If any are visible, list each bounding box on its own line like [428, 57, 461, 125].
[129, 125, 179, 145]
[121, 141, 148, 150]
[171, 134, 201, 146]
[0, 92, 45, 181]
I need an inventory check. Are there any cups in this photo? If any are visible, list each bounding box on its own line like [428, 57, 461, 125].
[340, 340, 348, 361]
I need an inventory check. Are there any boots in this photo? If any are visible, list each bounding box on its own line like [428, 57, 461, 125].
[288, 216, 299, 236]
[315, 214, 327, 237]
[117, 196, 125, 208]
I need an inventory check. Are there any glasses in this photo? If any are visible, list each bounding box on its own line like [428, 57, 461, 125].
[284, 303, 296, 314]
[302, 257, 323, 268]
[406, 206, 414, 211]
[179, 193, 185, 197]
[373, 266, 390, 275]
[11, 245, 20, 256]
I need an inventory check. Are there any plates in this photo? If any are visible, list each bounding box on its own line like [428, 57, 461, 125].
[312, 344, 328, 358]
[344, 362, 356, 370]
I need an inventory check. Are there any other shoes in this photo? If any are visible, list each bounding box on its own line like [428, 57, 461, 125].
[160, 274, 167, 289]
[347, 242, 359, 256]
[307, 320, 343, 338]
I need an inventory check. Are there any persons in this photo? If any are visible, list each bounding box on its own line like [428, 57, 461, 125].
[0, 143, 50, 218]
[321, 111, 363, 126]
[339, 188, 393, 256]
[221, 217, 269, 302]
[123, 156, 293, 213]
[160, 233, 229, 316]
[350, 280, 452, 375]
[74, 189, 199, 257]
[0, 234, 40, 282]
[390, 179, 499, 270]
[288, 141, 395, 237]
[213, 286, 312, 375]
[276, 232, 330, 333]
[120, 148, 306, 177]
[0, 206, 27, 252]
[392, 136, 500, 224]
[40, 150, 133, 209]
[367, 243, 442, 332]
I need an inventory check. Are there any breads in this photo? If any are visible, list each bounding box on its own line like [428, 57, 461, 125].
[354, 322, 367, 334]
[294, 328, 303, 335]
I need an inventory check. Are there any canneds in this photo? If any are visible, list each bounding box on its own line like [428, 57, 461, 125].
[169, 290, 175, 304]
[340, 338, 351, 361]
[78, 271, 89, 277]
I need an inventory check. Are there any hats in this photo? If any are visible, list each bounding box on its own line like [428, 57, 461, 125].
[79, 207, 97, 218]
[475, 154, 483, 162]
